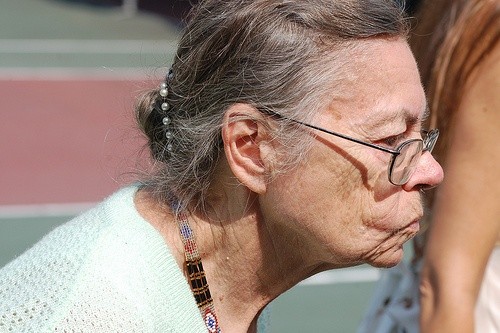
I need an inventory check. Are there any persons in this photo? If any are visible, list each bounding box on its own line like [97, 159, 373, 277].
[1, 1, 447, 333]
[360, 0, 500, 333]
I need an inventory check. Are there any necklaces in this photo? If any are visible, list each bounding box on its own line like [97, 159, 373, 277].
[170, 195, 225, 333]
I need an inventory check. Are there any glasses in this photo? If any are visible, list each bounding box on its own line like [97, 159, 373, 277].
[261, 106, 442, 186]
